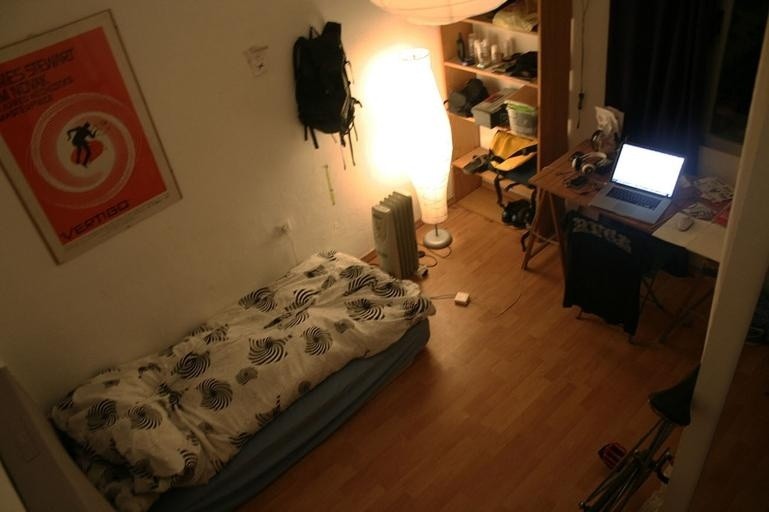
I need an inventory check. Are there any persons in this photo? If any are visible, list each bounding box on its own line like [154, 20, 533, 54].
[65, 120, 101, 169]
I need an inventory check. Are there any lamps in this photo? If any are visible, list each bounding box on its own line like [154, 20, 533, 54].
[400, 48, 454, 249]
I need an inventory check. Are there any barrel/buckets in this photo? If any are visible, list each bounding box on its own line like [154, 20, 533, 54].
[505, 107, 537, 135]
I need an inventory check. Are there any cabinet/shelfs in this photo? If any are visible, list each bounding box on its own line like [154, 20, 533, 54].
[440, 0, 572, 241]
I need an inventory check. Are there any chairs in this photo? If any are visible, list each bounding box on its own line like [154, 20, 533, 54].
[560, 210, 664, 344]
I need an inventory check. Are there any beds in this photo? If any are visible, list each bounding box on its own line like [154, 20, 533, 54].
[47, 248, 436, 512]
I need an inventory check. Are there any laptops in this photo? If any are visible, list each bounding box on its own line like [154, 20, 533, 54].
[589, 139, 688, 226]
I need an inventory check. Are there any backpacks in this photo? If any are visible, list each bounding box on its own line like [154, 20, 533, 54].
[292, 20, 355, 134]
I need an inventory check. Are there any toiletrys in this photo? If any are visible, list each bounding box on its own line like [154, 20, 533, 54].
[457, 32, 514, 65]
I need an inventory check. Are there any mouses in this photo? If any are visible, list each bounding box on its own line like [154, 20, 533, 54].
[677, 216, 695, 231]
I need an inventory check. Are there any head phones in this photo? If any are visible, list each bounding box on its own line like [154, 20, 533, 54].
[571, 151, 608, 176]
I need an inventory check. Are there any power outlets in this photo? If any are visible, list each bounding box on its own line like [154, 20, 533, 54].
[276, 217, 292, 238]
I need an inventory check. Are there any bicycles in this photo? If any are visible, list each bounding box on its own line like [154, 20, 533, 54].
[579, 362, 701, 510]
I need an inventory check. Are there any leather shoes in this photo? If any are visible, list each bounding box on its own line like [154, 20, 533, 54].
[502, 198, 534, 227]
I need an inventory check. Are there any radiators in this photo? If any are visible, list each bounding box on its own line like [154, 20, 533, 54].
[371, 190, 420, 279]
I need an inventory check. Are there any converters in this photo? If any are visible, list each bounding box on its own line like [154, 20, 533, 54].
[454, 292, 471, 306]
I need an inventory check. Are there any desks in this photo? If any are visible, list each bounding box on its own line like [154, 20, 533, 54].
[525, 136, 769, 344]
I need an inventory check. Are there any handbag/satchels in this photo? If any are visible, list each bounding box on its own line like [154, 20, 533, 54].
[449, 78, 487, 117]
[488, 131, 538, 186]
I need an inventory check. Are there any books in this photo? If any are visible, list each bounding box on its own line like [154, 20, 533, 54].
[502, 84, 538, 111]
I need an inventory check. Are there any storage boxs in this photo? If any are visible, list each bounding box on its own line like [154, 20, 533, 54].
[507, 106, 538, 138]
